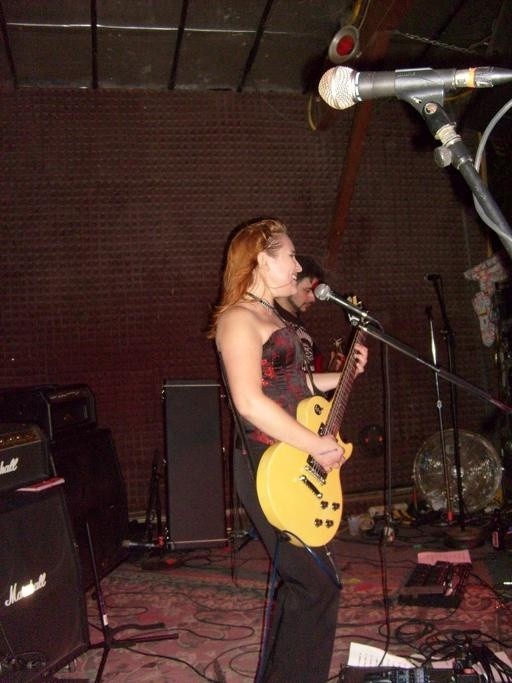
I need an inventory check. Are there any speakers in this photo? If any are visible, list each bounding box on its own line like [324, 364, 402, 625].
[161, 377, 229, 552]
[46, 427, 134, 578]
[1, 483, 88, 683]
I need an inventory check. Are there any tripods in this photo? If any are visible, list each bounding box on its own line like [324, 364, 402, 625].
[80, 520, 179, 681]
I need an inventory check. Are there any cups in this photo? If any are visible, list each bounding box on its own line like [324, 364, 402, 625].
[348, 513, 359, 534]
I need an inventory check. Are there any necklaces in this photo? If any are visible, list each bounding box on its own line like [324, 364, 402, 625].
[246, 289, 276, 311]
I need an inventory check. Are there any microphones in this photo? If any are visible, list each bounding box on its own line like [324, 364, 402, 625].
[318, 63, 512, 110]
[314, 283, 379, 328]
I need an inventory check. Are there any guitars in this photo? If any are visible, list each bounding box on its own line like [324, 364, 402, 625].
[256, 297, 371, 548]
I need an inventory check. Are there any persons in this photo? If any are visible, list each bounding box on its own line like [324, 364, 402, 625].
[204, 218, 371, 680]
[270, 249, 347, 399]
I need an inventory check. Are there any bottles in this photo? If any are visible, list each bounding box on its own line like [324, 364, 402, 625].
[491, 507, 502, 551]
[383, 513, 394, 545]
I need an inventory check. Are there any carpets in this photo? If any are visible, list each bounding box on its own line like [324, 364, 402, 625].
[52, 513, 512, 682]
